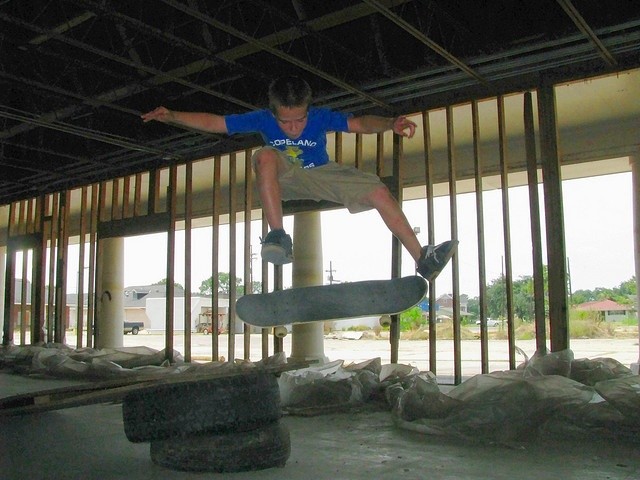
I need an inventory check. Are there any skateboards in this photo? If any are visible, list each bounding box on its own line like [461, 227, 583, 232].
[235, 276, 428, 339]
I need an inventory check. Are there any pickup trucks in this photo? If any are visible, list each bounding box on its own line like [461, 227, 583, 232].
[91, 317, 145, 338]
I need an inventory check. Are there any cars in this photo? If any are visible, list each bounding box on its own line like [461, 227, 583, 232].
[200, 324, 225, 335]
[475, 317, 501, 327]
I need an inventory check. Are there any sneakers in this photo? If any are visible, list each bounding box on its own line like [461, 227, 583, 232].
[416, 238, 459, 283]
[259, 228, 294, 264]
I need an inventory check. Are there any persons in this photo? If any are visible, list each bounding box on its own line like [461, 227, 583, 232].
[140, 75, 459, 283]
